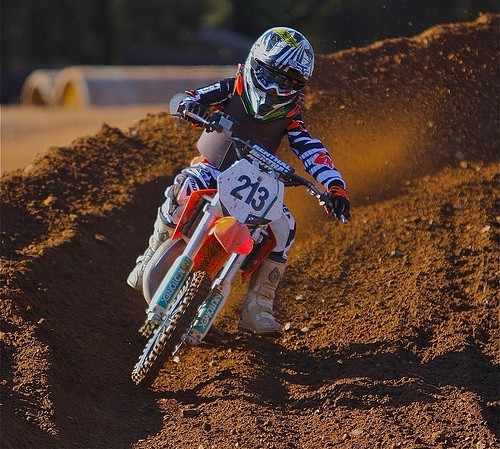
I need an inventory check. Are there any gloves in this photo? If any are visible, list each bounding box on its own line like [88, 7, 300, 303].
[323, 186, 351, 221]
[178, 99, 210, 128]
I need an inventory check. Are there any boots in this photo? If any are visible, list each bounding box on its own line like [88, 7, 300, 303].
[237, 258, 287, 337]
[126, 206, 177, 291]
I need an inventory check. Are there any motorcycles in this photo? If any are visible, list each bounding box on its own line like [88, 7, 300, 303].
[125, 105, 350, 387]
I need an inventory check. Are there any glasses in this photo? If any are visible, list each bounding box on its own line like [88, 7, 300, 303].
[250, 59, 309, 98]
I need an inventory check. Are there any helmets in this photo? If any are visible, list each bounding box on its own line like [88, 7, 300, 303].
[243, 27, 315, 122]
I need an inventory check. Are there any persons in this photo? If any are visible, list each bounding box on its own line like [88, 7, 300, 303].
[126, 27, 350, 335]
